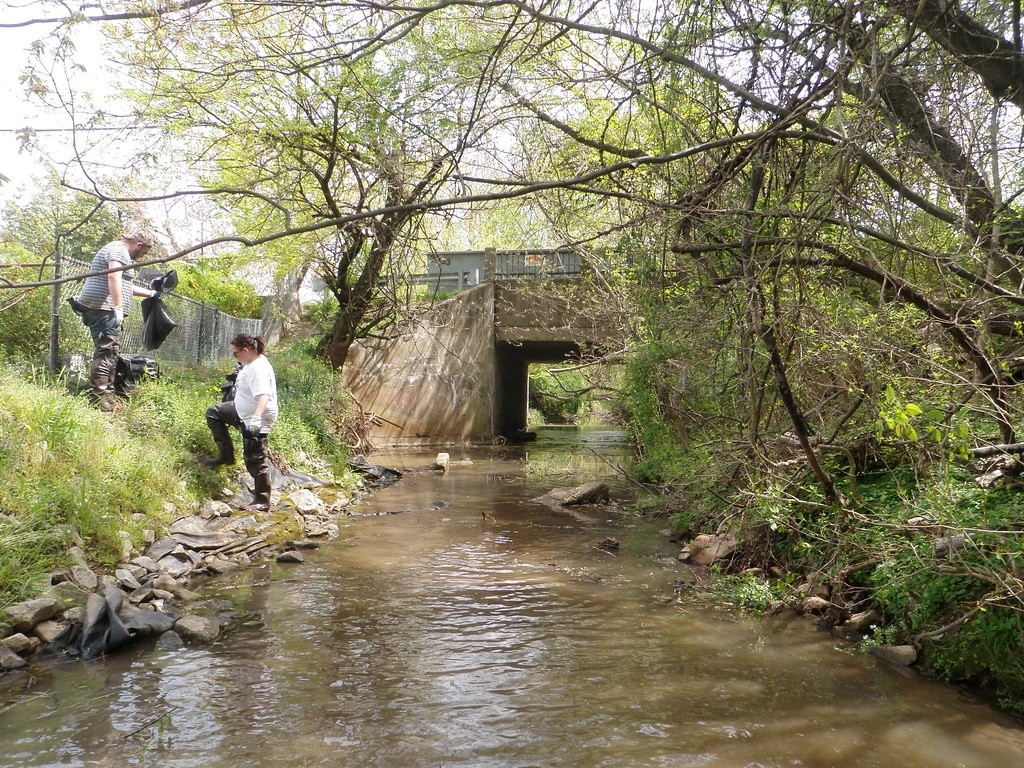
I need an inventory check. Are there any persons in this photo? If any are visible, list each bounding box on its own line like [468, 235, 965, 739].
[204, 334, 279, 505]
[76, 227, 153, 412]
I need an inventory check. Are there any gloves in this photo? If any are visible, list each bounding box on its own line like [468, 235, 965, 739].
[114, 304, 123, 329]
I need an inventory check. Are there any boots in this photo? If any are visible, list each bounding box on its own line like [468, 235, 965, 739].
[89, 334, 121, 411]
[239, 438, 270, 511]
[206, 401, 234, 467]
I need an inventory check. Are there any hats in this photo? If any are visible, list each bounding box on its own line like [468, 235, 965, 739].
[121, 229, 153, 248]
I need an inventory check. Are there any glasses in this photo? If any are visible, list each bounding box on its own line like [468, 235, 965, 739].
[233, 350, 243, 358]
